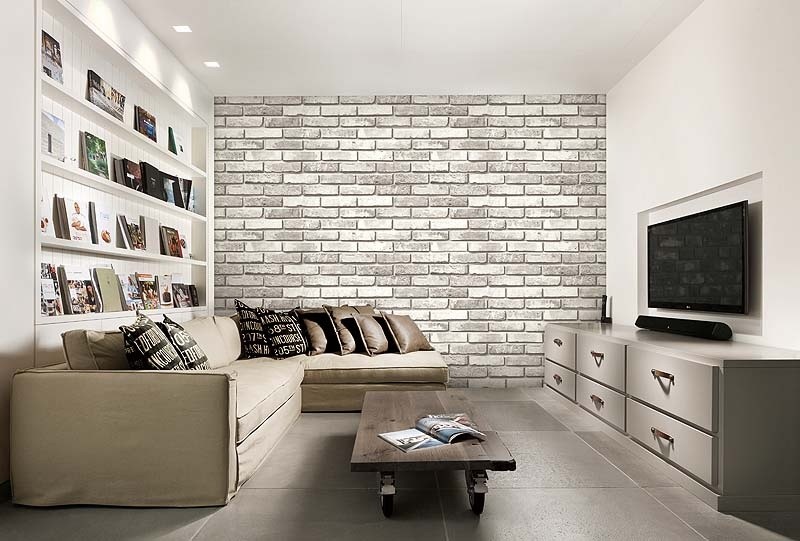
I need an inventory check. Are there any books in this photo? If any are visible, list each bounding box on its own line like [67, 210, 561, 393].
[41, 31, 63, 84]
[377, 418, 487, 454]
[115, 158, 194, 211]
[136, 106, 157, 143]
[169, 128, 188, 160]
[88, 70, 126, 122]
[41, 109, 66, 162]
[54, 193, 183, 258]
[41, 261, 199, 317]
[82, 131, 110, 180]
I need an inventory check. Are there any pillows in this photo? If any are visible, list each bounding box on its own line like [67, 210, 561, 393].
[234, 299, 275, 360]
[180, 317, 234, 369]
[351, 313, 400, 357]
[295, 309, 340, 356]
[322, 304, 376, 356]
[156, 314, 212, 370]
[213, 313, 247, 362]
[255, 306, 312, 360]
[118, 311, 186, 370]
[380, 311, 435, 354]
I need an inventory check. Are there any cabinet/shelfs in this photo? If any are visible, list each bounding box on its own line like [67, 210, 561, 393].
[34, 0, 215, 368]
[542, 322, 800, 512]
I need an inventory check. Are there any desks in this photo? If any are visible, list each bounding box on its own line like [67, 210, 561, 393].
[351, 391, 517, 518]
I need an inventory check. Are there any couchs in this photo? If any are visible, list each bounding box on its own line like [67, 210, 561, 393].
[9, 310, 449, 507]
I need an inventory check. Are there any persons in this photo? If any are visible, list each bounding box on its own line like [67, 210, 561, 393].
[72, 202, 88, 231]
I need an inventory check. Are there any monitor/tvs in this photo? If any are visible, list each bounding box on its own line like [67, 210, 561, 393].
[647, 200, 749, 315]
